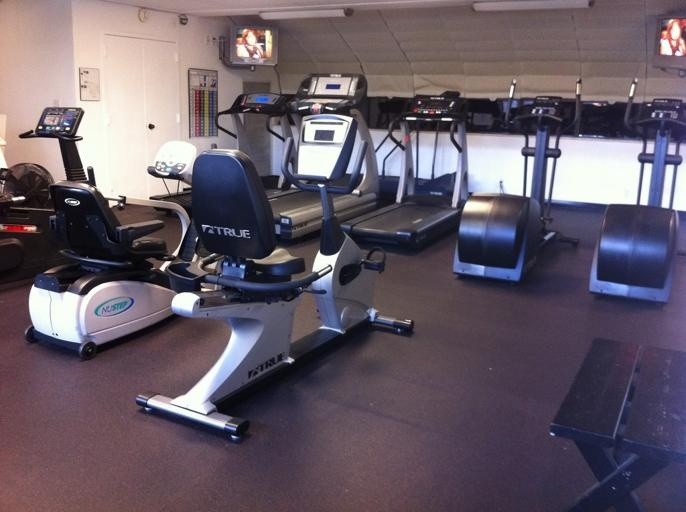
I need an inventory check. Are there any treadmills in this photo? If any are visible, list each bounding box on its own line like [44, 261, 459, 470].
[340, 91, 468, 255]
[150, 75, 379, 241]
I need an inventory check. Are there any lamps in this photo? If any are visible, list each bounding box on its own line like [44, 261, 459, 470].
[259, 8, 354, 20]
[470, 0, 594, 21]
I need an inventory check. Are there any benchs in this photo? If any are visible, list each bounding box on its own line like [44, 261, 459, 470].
[549, 337, 686, 512]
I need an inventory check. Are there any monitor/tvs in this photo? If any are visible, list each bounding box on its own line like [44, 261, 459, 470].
[653, 14, 686, 71]
[223, 24, 278, 68]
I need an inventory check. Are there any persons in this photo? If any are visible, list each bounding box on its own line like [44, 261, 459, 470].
[657, 19, 686, 56]
[235, 29, 266, 59]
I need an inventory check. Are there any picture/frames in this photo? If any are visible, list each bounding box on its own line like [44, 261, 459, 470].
[187, 68, 218, 139]
[79, 67, 101, 100]
